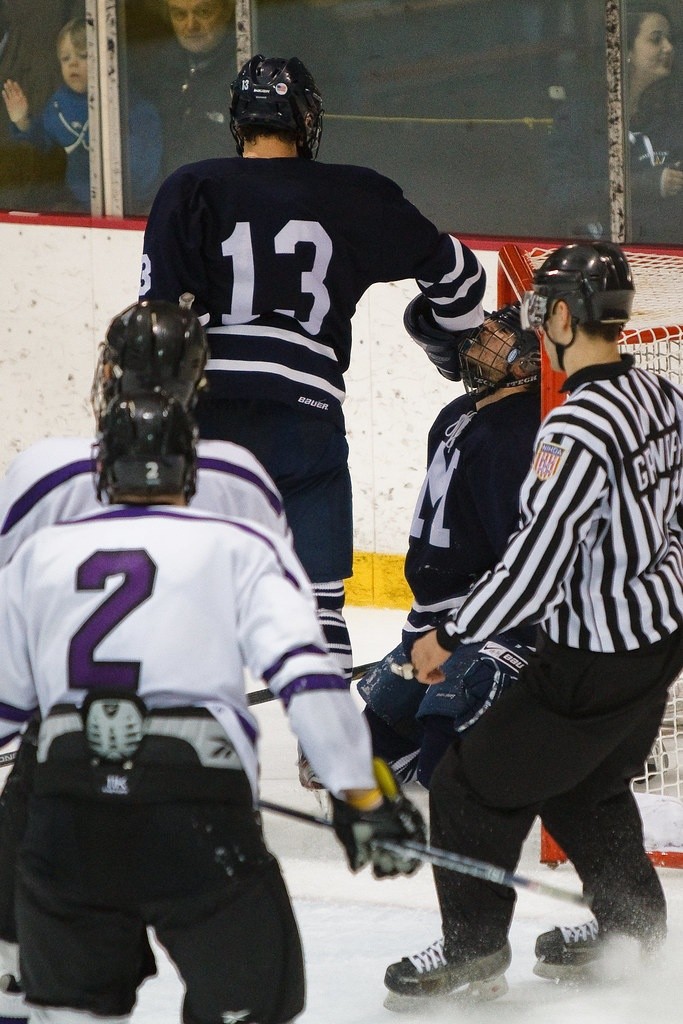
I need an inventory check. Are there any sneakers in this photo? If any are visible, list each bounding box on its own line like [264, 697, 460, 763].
[535, 891, 666, 982]
[384, 919, 511, 1011]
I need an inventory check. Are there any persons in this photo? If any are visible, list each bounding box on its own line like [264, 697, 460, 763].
[386, 238, 683, 1015]
[540, 8, 683, 245]
[2, 17, 163, 213]
[358, 302, 540, 790]
[0, 302, 424, 1024]
[151, 0, 234, 175]
[141, 56, 484, 789]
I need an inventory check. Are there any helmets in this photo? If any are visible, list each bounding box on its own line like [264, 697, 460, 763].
[230, 54, 325, 160]
[93, 300, 208, 504]
[452, 302, 541, 401]
[528, 240, 635, 349]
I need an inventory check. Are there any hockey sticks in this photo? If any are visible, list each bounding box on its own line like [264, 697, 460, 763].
[242, 662, 384, 705]
[257, 799, 596, 909]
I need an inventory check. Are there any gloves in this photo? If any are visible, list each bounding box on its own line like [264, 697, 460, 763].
[329, 757, 426, 877]
[404, 293, 482, 382]
